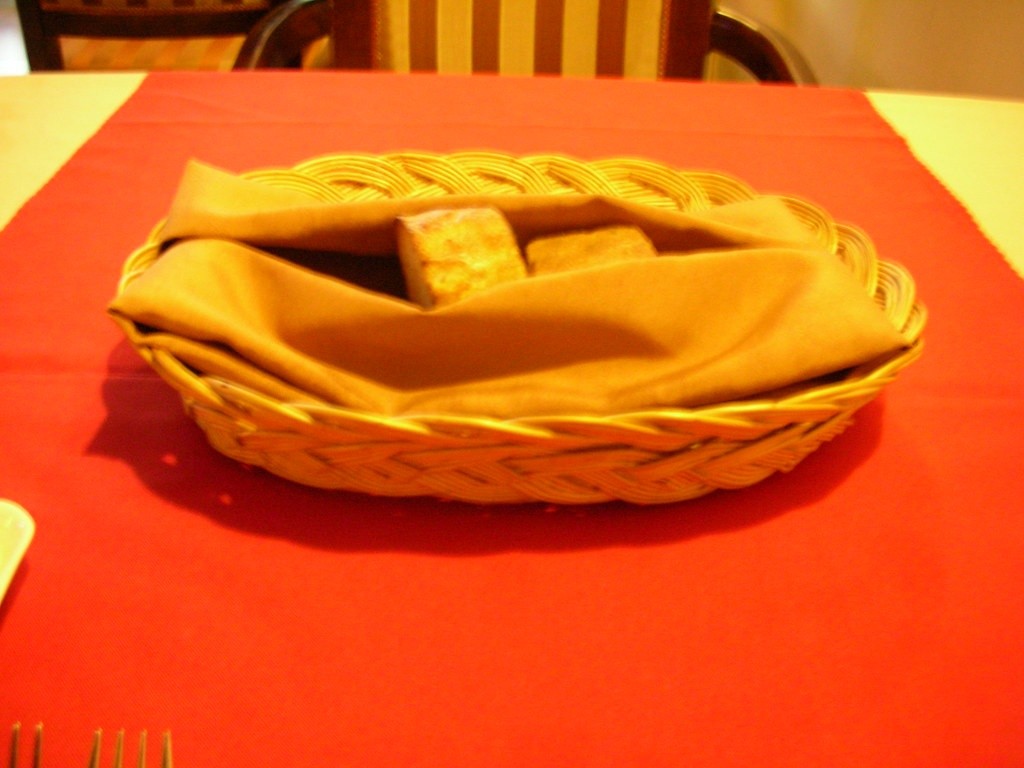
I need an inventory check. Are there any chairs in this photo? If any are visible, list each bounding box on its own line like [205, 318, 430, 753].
[231, 0, 823, 88]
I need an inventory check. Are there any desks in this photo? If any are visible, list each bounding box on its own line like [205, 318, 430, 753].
[0, 71, 1024, 768]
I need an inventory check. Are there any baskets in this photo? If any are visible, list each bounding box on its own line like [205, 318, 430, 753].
[119, 153, 927, 503]
